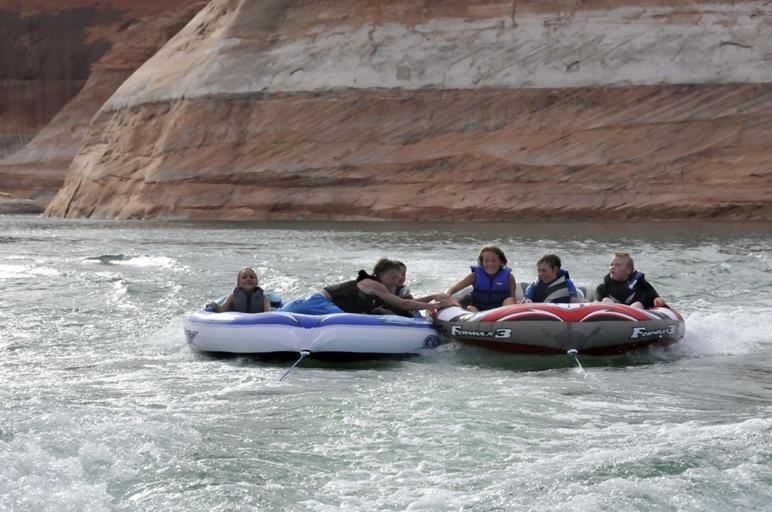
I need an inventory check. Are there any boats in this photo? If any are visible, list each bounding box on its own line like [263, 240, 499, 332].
[187, 294, 437, 357]
[433, 281, 684, 351]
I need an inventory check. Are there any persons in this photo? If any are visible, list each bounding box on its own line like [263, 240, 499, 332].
[280, 258, 462, 319]
[520, 253, 580, 305]
[593, 251, 664, 310]
[440, 247, 516, 314]
[204, 268, 275, 313]
[371, 260, 452, 321]
